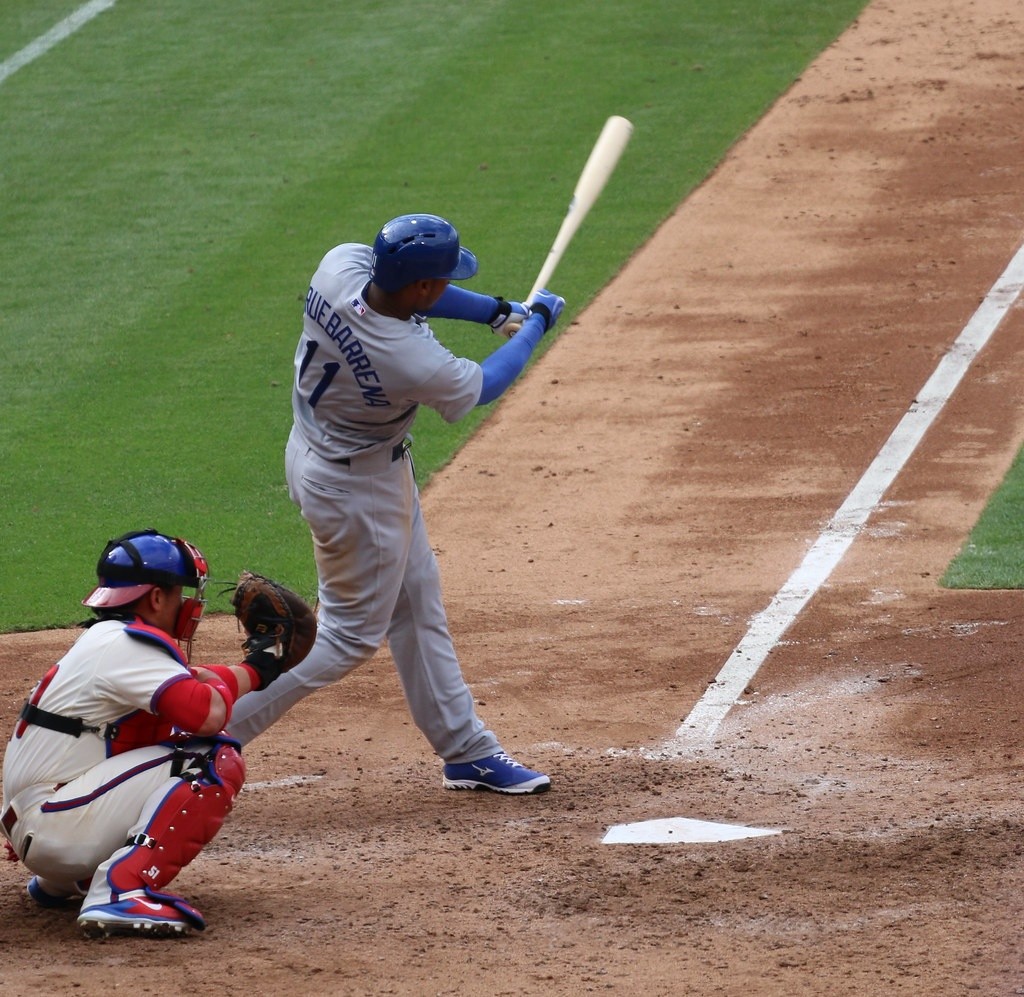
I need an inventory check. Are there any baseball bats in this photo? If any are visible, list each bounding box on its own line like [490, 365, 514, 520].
[502, 112, 637, 342]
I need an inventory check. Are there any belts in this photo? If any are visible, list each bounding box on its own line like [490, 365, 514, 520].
[335, 438, 407, 466]
[2, 784, 67, 837]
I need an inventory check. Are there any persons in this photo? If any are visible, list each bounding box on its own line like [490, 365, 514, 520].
[0, 527, 290, 939]
[223, 215, 566, 799]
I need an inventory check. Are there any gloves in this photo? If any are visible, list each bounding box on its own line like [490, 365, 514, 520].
[489, 302, 531, 337]
[528, 289, 566, 334]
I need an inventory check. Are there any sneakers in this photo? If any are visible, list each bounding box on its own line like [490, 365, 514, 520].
[443, 752, 550, 795]
[78, 885, 191, 940]
[27, 876, 92, 906]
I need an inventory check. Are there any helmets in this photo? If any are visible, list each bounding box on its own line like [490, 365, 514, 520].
[79, 530, 184, 607]
[368, 214, 478, 291]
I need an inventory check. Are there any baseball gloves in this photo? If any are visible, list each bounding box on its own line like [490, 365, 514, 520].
[214, 566, 320, 694]
